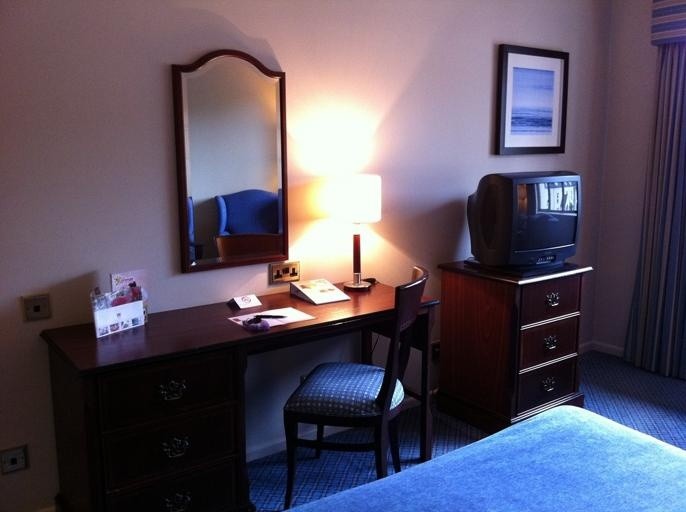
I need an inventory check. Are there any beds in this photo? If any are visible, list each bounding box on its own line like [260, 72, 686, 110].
[279, 404, 686, 512]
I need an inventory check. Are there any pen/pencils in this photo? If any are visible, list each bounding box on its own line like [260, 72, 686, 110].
[255, 315, 286, 318]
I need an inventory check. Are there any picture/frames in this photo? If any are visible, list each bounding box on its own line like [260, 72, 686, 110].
[493, 43, 570, 156]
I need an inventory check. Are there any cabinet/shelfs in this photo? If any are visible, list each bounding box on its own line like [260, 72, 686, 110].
[435, 262, 595, 437]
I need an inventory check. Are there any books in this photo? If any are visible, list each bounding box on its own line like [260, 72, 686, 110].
[110, 269, 144, 306]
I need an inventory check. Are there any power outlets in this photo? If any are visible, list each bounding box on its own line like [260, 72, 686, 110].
[271, 261, 301, 283]
[0, 446, 31, 473]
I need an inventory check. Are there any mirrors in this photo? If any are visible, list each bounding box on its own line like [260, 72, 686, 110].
[171, 48, 289, 273]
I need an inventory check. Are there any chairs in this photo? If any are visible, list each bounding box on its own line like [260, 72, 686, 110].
[282, 266, 428, 511]
[188, 197, 195, 262]
[216, 190, 282, 236]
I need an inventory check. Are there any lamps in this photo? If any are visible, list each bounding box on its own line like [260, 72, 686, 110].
[332, 174, 382, 292]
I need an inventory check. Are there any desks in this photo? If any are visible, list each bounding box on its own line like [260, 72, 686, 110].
[205, 233, 285, 262]
[39, 280, 440, 510]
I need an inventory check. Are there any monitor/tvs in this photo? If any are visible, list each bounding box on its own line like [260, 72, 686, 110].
[467, 171, 583, 276]
